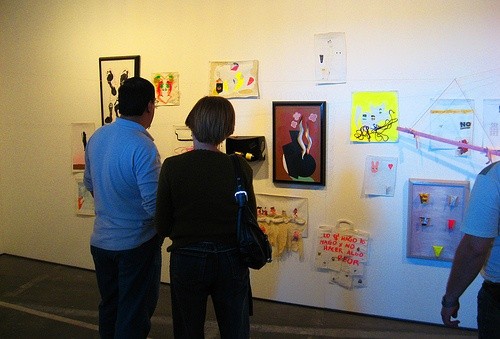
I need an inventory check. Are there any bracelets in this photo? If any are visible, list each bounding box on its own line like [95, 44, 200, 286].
[442, 295, 459, 307]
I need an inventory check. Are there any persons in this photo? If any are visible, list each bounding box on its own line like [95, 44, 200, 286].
[83, 76, 162, 339]
[441, 161, 500, 339]
[155, 95, 258, 339]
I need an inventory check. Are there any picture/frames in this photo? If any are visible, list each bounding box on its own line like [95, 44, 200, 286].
[271, 100, 326, 187]
[405, 178, 470, 262]
[99, 56, 140, 126]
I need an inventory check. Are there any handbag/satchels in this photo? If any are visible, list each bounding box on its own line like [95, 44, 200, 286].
[230, 154, 272, 271]
